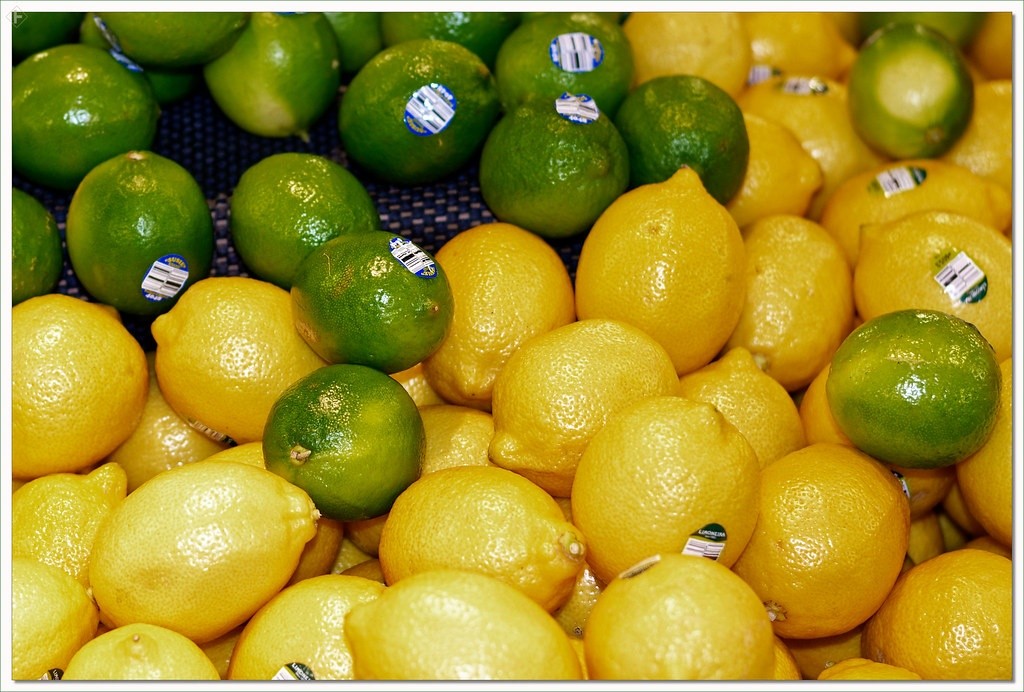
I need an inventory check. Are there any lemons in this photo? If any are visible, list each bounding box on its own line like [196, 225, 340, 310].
[12, 12, 1013, 681]
